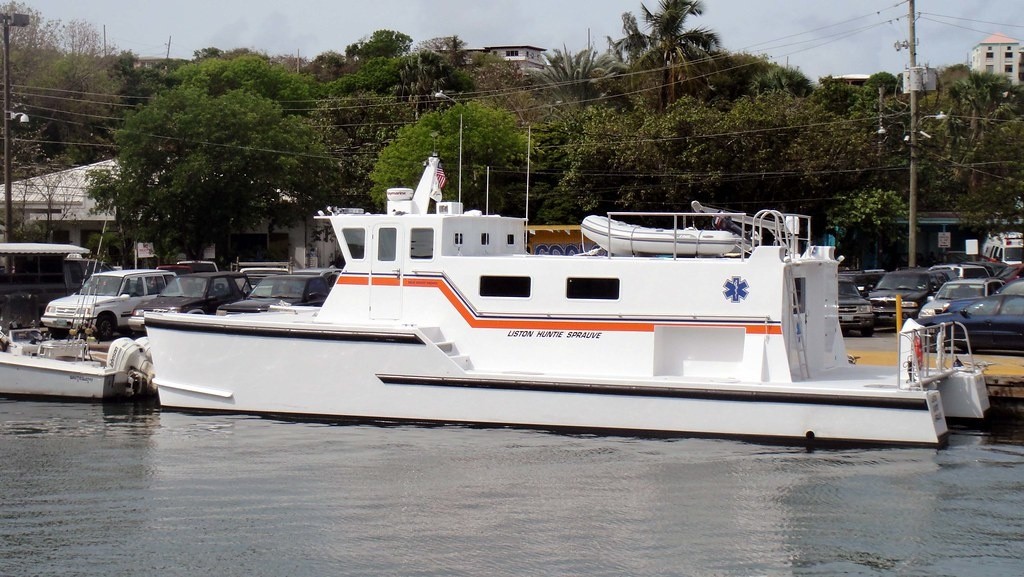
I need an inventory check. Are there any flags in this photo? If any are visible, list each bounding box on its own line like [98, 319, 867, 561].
[435, 163, 447, 189]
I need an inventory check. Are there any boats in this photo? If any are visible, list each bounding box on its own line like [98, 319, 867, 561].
[1, 327, 141, 404]
[147, 114, 994, 450]
[580, 214, 743, 258]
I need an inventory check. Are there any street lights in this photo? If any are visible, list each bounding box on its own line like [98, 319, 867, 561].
[436, 93, 464, 216]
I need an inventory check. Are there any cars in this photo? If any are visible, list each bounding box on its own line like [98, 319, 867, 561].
[868, 266, 949, 330]
[912, 278, 1024, 354]
[143, 256, 345, 286]
[838, 277, 875, 338]
[128, 271, 250, 334]
[919, 276, 1007, 317]
[39, 269, 177, 340]
[217, 276, 331, 317]
[845, 230, 1024, 291]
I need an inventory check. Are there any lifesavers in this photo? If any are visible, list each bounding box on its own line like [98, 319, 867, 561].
[913, 337, 923, 370]
